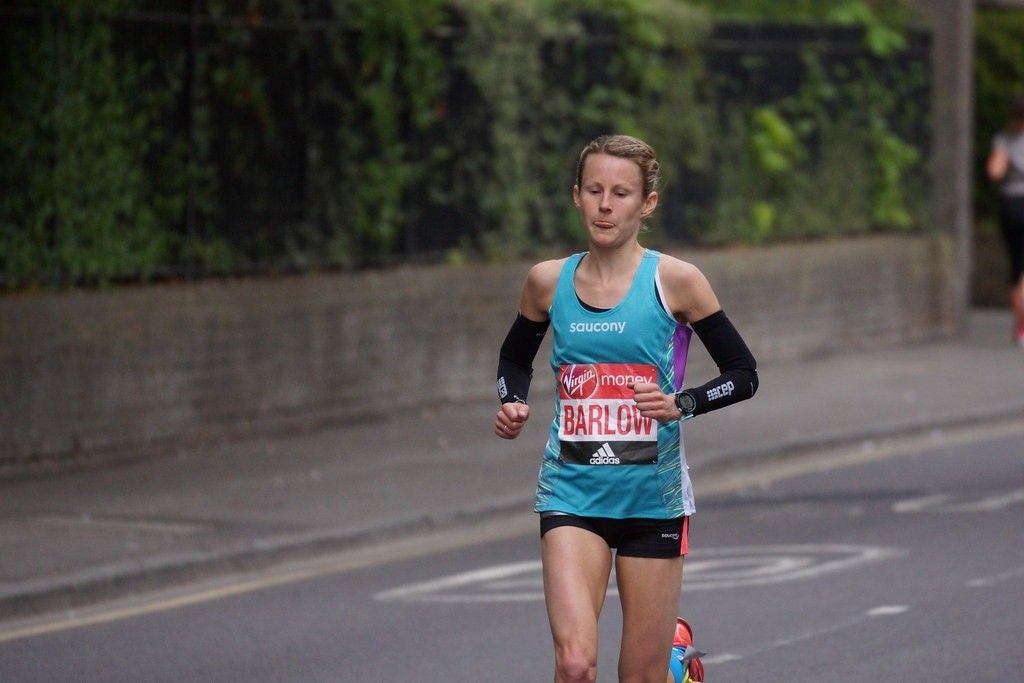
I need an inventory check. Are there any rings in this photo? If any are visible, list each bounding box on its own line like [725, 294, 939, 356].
[504, 426, 506, 430]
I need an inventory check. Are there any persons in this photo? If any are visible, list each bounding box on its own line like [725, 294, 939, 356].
[494, 134, 759, 683]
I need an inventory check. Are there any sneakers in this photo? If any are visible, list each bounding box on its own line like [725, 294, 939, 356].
[672, 617, 704, 683]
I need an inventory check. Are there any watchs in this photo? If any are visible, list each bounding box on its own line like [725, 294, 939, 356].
[675, 392, 696, 421]
